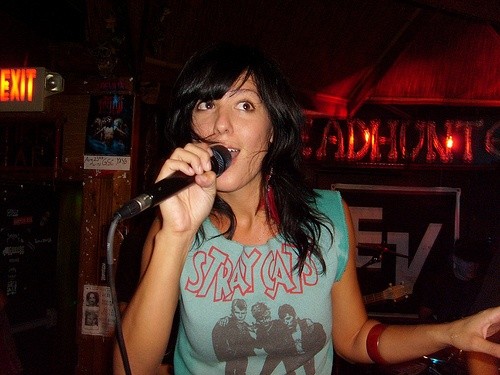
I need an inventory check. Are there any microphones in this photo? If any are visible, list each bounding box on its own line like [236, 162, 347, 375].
[112, 145, 232, 221]
